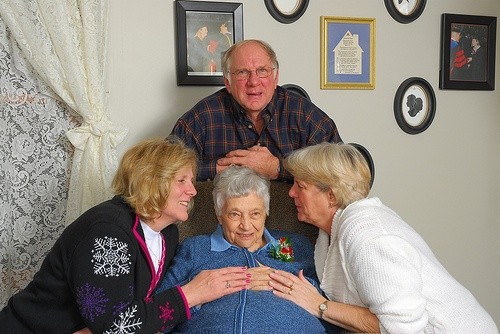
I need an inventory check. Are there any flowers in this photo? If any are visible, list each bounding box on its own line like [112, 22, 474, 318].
[266, 236, 295, 264]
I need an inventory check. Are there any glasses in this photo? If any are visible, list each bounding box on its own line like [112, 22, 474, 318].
[228, 66, 276, 81]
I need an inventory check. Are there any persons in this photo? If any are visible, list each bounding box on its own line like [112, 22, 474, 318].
[0, 140, 252, 334]
[172, 38, 343, 183]
[268, 142, 499, 334]
[450, 29, 487, 80]
[148, 167, 329, 334]
[189, 21, 232, 72]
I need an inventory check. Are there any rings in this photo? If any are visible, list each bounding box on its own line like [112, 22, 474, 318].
[288, 289, 292, 294]
[290, 283, 294, 287]
[226, 281, 230, 288]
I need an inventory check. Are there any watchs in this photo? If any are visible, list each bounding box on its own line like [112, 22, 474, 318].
[319, 300, 328, 318]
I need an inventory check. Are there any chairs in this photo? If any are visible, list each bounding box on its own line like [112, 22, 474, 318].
[176, 174, 319, 247]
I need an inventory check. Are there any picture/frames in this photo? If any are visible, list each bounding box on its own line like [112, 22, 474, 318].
[318, 17, 382, 94]
[394, 74, 437, 136]
[263, 0, 312, 24]
[438, 10, 500, 96]
[170, 0, 247, 92]
[382, 0, 430, 24]
